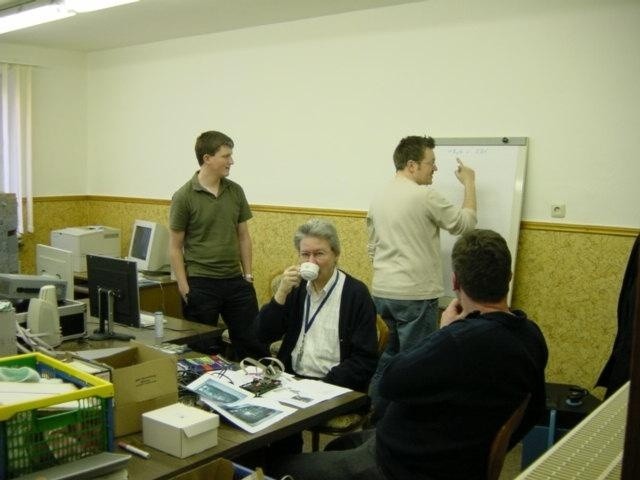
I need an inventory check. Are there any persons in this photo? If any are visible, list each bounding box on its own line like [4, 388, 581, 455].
[280, 224, 551, 480]
[362, 134, 478, 428]
[169, 129, 271, 370]
[229, 213, 380, 480]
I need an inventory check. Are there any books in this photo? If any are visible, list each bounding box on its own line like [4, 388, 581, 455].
[0, 380, 100, 411]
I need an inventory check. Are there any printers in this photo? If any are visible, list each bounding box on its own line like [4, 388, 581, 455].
[50, 226, 121, 274]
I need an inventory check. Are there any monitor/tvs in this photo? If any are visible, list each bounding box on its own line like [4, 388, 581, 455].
[35, 243, 74, 301]
[127, 219, 170, 279]
[86, 253, 140, 341]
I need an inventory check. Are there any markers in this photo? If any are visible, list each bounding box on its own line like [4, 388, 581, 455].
[457, 163, 460, 172]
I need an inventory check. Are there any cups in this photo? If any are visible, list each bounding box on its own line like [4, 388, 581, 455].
[298, 262, 320, 280]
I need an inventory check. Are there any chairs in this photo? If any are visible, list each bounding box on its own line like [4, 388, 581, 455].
[301, 309, 390, 454]
[482, 385, 541, 480]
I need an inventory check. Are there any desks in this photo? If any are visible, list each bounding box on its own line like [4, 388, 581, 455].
[74, 307, 227, 353]
[75, 269, 185, 319]
[50, 343, 376, 480]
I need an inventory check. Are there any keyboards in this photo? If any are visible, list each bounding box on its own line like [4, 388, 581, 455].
[140, 312, 168, 328]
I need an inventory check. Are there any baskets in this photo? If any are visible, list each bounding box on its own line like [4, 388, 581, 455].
[0, 352, 116, 479]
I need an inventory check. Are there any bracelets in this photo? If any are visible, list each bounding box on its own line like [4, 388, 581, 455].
[242, 271, 255, 283]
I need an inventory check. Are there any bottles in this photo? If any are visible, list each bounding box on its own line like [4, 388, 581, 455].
[154, 311, 164, 339]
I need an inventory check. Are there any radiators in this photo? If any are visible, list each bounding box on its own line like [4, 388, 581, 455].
[514, 378, 629, 480]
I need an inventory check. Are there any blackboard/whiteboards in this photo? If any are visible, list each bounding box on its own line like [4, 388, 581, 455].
[419, 137, 529, 310]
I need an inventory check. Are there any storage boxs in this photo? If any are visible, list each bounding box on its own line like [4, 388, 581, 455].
[65, 337, 182, 438]
[140, 401, 222, 462]
